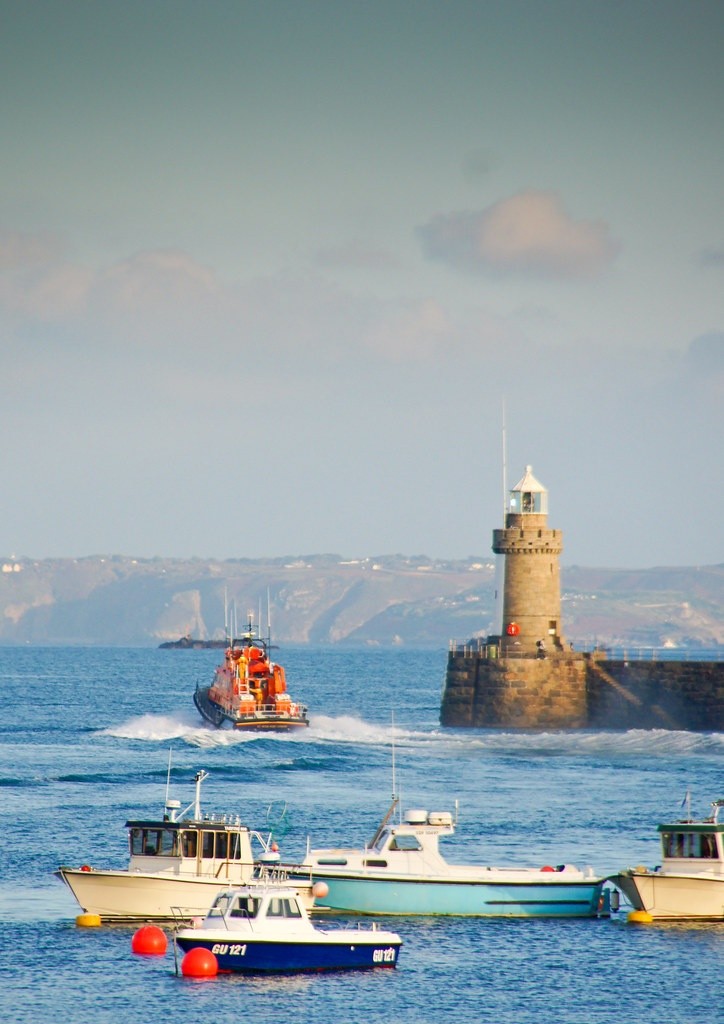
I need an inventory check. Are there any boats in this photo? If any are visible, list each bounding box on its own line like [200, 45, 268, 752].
[57, 745, 331, 922]
[171, 796, 403, 968]
[593, 789, 724, 926]
[192, 583, 310, 733]
[269, 704, 611, 921]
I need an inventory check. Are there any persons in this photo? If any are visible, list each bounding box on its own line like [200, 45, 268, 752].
[537, 638, 548, 660]
[570, 642, 575, 652]
[249, 684, 263, 710]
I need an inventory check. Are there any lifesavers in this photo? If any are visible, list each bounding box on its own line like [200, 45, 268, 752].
[507, 624, 520, 636]
[287, 703, 299, 716]
